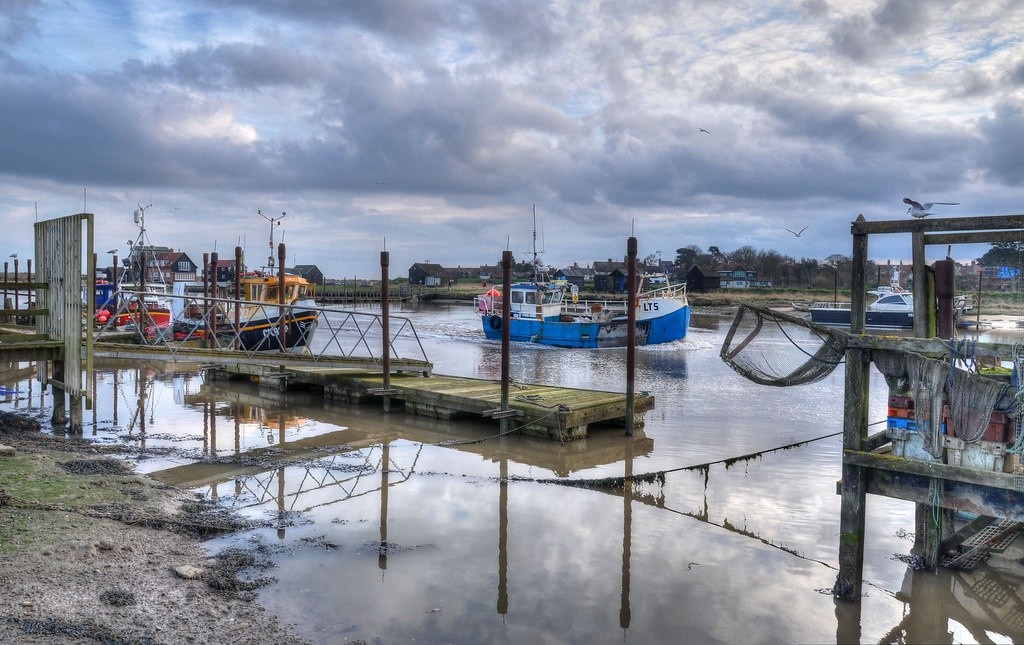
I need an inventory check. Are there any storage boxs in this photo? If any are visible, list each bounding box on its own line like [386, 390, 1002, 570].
[885, 427, 943, 462]
[884, 416, 945, 433]
[945, 437, 1006, 472]
[888, 406, 945, 422]
[943, 404, 1006, 441]
[888, 395, 916, 408]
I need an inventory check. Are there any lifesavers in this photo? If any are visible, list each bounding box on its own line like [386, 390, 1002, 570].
[489, 314, 501, 330]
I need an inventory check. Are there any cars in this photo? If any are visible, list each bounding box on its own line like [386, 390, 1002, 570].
[359, 282, 374, 288]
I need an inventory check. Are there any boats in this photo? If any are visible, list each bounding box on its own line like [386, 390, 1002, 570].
[97, 202, 169, 334]
[810, 265, 979, 330]
[172, 210, 318, 354]
[473, 203, 690, 349]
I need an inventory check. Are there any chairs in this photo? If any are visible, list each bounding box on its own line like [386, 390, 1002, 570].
[590, 302, 603, 317]
